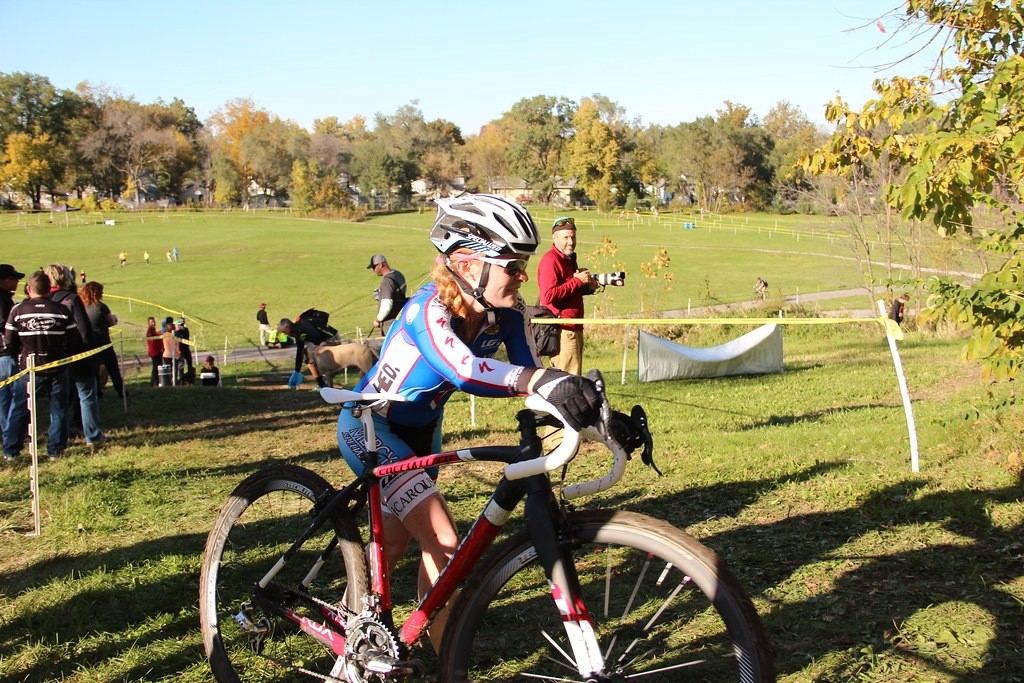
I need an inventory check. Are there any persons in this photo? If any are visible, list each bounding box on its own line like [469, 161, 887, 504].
[146, 316, 196, 388]
[366, 254, 407, 337]
[321, 194, 604, 655]
[276, 318, 342, 393]
[167, 246, 179, 263]
[144, 251, 150, 265]
[200, 356, 219, 387]
[753, 277, 766, 301]
[888, 294, 910, 324]
[1, 263, 129, 461]
[537, 217, 607, 443]
[119, 250, 128, 267]
[257, 303, 294, 348]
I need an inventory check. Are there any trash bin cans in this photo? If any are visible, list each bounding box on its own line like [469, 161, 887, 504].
[684, 223, 689, 228]
[690, 223, 694, 229]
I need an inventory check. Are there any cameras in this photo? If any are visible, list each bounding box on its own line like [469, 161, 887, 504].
[578, 268, 625, 294]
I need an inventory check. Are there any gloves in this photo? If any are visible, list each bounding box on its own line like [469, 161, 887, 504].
[516, 367, 600, 432]
[289, 371, 298, 388]
[373, 320, 379, 328]
[373, 288, 380, 300]
[612, 419, 631, 444]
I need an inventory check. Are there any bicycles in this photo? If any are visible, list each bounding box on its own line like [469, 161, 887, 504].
[195, 367, 778, 683]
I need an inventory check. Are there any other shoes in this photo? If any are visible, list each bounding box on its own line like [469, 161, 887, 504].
[308, 385, 321, 393]
[414, 636, 441, 674]
[50, 449, 64, 461]
[87, 438, 109, 452]
[4, 453, 23, 464]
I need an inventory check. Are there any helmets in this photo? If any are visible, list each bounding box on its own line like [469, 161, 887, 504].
[429, 194, 540, 255]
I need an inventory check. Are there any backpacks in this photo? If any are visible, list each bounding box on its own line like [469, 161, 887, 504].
[296, 308, 329, 333]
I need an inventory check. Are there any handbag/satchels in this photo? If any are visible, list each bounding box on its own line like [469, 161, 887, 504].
[400, 298, 408, 307]
[526, 305, 559, 356]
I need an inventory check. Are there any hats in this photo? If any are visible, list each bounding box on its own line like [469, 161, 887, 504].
[552, 217, 577, 234]
[205, 356, 213, 362]
[260, 303, 266, 307]
[366, 255, 386, 269]
[0, 264, 25, 280]
[173, 321, 182, 325]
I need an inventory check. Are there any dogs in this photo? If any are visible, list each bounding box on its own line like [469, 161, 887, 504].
[303, 342, 380, 388]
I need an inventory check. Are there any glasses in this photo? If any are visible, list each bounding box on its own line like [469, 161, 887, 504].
[371, 265, 378, 269]
[453, 252, 528, 276]
[552, 218, 574, 230]
